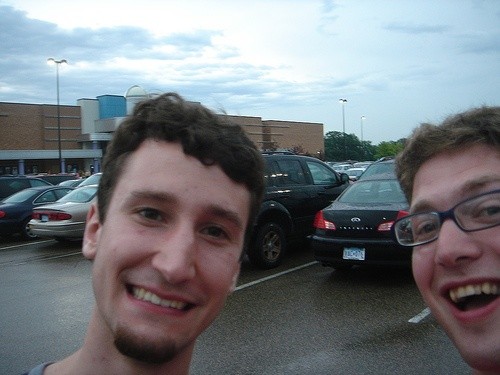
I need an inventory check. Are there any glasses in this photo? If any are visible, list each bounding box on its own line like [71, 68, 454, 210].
[388, 189, 500, 248]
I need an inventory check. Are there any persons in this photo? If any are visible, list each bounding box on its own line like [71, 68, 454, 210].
[18, 93, 269, 375]
[391, 107, 500, 375]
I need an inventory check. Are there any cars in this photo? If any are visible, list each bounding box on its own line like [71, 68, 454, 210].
[28, 183, 99, 245]
[0, 185, 79, 241]
[0, 171, 104, 191]
[317, 159, 375, 183]
[310, 157, 413, 269]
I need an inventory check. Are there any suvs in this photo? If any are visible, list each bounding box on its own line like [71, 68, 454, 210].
[243, 149, 354, 270]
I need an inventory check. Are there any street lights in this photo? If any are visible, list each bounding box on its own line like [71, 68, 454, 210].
[360, 115, 365, 162]
[339, 99, 348, 160]
[47, 57, 70, 173]
